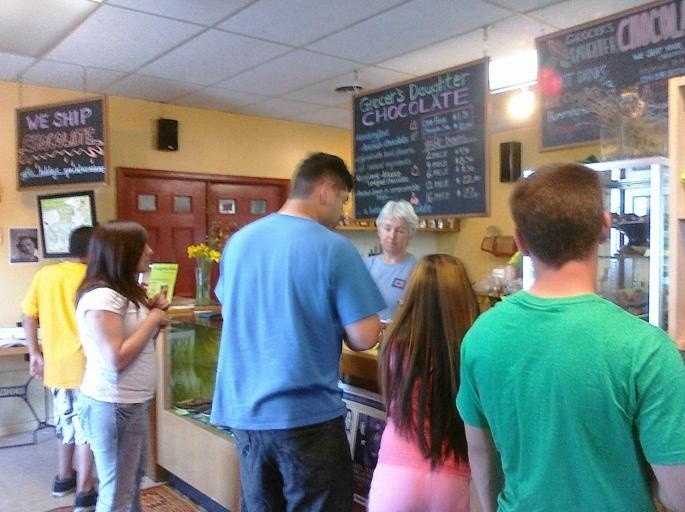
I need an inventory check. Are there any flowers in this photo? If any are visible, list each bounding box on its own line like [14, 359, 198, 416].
[186, 204, 238, 304]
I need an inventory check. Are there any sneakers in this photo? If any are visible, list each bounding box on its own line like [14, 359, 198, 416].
[73, 487, 99, 512]
[51, 469, 77, 497]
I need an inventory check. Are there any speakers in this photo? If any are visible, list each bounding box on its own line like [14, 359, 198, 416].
[500, 140, 519, 184]
[157, 116, 178, 151]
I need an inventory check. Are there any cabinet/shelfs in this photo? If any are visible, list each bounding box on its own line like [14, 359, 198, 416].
[157, 305, 241, 506]
[522, 156, 668, 335]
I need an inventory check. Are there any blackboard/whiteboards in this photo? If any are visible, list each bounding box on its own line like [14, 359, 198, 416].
[349, 55, 491, 222]
[533, 0, 685, 152]
[15, 93, 109, 193]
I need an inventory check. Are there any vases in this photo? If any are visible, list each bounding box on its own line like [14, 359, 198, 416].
[600, 117, 664, 159]
[196, 264, 210, 306]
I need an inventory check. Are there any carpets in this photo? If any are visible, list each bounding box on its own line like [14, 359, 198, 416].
[45, 483, 201, 512]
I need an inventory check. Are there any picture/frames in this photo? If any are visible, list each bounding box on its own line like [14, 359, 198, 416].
[7, 226, 41, 265]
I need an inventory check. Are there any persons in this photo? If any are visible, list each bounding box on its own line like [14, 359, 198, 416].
[365, 200, 419, 323]
[368, 253, 481, 512]
[20, 226, 99, 512]
[456, 161, 685, 512]
[210, 151, 389, 512]
[15, 233, 38, 262]
[505, 249, 523, 282]
[76, 219, 171, 512]
